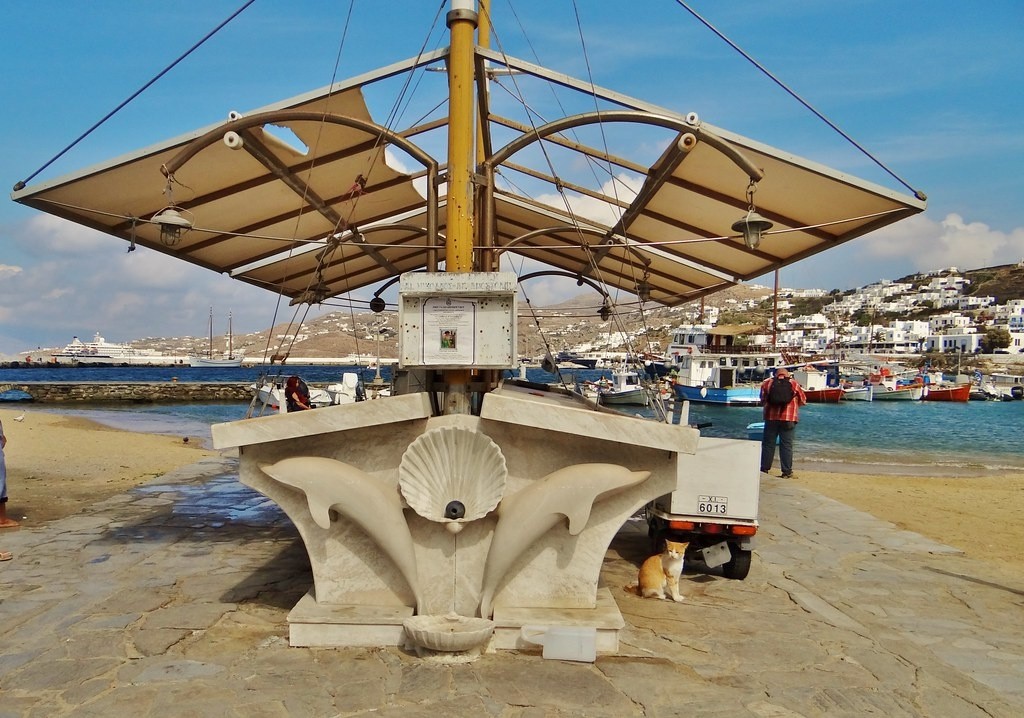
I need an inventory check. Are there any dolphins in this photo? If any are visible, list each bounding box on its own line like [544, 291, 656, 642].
[480, 462, 652, 619]
[258, 456, 431, 615]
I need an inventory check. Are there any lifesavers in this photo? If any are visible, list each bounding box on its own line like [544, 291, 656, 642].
[688, 347, 692, 354]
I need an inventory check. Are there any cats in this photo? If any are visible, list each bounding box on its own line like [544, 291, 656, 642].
[624, 539, 691, 602]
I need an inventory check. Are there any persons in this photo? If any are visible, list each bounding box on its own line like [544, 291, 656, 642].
[284, 376, 311, 411]
[759, 369, 807, 479]
[0, 419, 20, 528]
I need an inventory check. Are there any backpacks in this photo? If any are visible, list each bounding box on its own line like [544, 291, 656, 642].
[768, 376, 793, 405]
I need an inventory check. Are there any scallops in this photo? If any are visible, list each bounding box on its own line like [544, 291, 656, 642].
[399, 426, 509, 533]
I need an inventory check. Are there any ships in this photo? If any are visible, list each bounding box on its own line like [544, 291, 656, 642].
[51, 332, 190, 366]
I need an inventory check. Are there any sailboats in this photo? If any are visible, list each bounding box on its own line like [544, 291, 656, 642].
[921, 381, 973, 403]
[828, 297, 873, 404]
[189, 306, 244, 368]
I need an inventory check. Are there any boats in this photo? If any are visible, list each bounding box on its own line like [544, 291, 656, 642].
[251, 381, 374, 410]
[868, 366, 928, 401]
[987, 373, 1024, 400]
[957, 352, 988, 400]
[678, 323, 793, 389]
[590, 352, 650, 407]
[670, 367, 773, 409]
[790, 365, 846, 404]
[637, 325, 714, 382]
